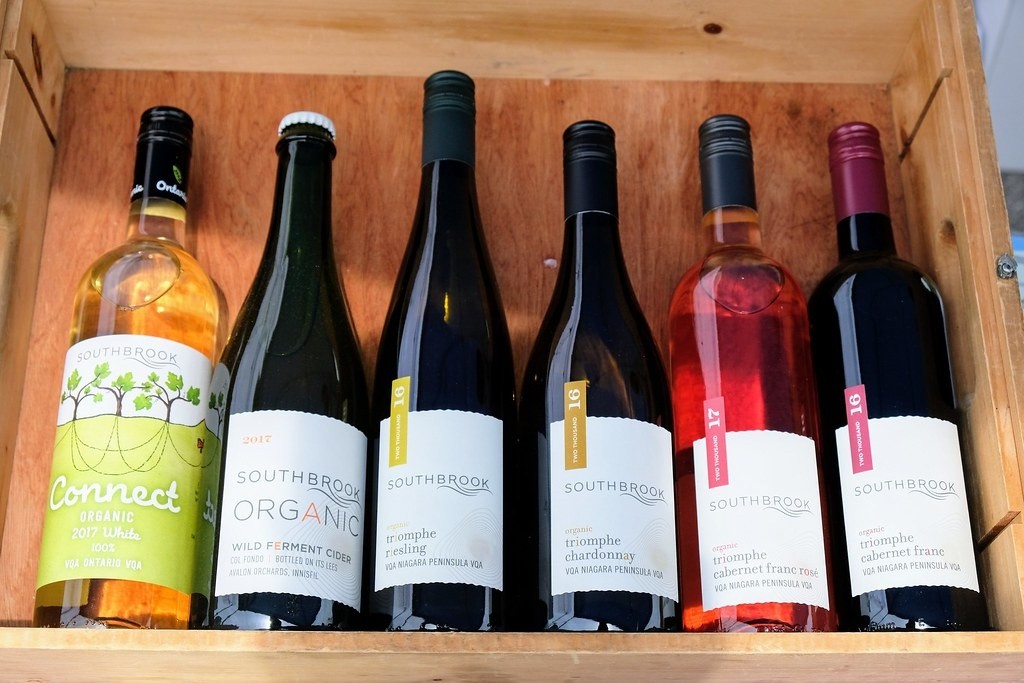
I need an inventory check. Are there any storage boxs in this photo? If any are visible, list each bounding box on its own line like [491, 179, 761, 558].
[0, 0, 1024, 683]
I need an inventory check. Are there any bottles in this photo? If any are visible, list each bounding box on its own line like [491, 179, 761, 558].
[207, 112, 368, 630]
[521, 119, 682, 632]
[32, 106, 228, 629]
[808, 121, 991, 631]
[362, 71, 527, 633]
[667, 113, 839, 632]
